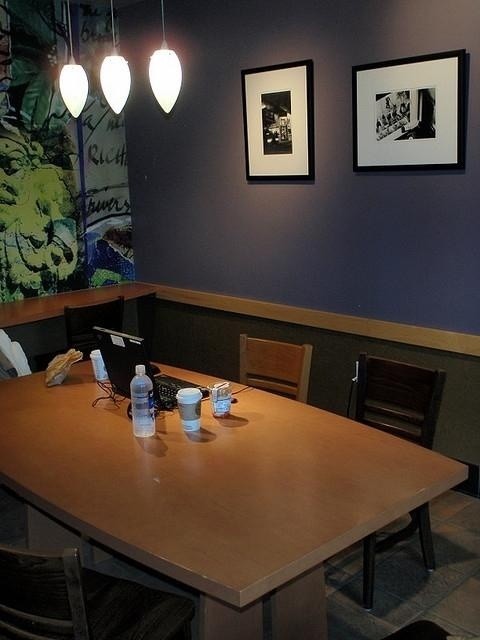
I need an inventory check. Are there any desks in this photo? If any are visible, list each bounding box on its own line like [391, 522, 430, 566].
[0, 355, 470, 640]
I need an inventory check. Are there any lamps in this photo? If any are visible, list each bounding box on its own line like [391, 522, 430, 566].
[100, 1, 133, 117]
[147, 0, 185, 115]
[58, 0, 91, 120]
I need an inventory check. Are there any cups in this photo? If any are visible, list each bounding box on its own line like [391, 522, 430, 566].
[207, 383, 232, 418]
[90, 349, 108, 381]
[175, 387, 204, 432]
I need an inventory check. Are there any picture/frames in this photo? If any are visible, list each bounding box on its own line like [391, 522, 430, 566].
[350, 48, 469, 172]
[239, 58, 317, 185]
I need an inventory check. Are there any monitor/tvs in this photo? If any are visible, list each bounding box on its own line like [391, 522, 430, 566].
[92, 326, 210, 411]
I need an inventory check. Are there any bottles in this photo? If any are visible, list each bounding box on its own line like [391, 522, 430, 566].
[130, 365, 156, 438]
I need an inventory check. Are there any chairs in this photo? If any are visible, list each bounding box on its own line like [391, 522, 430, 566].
[326, 351, 448, 611]
[238, 332, 314, 404]
[63, 294, 127, 363]
[0, 540, 197, 639]
